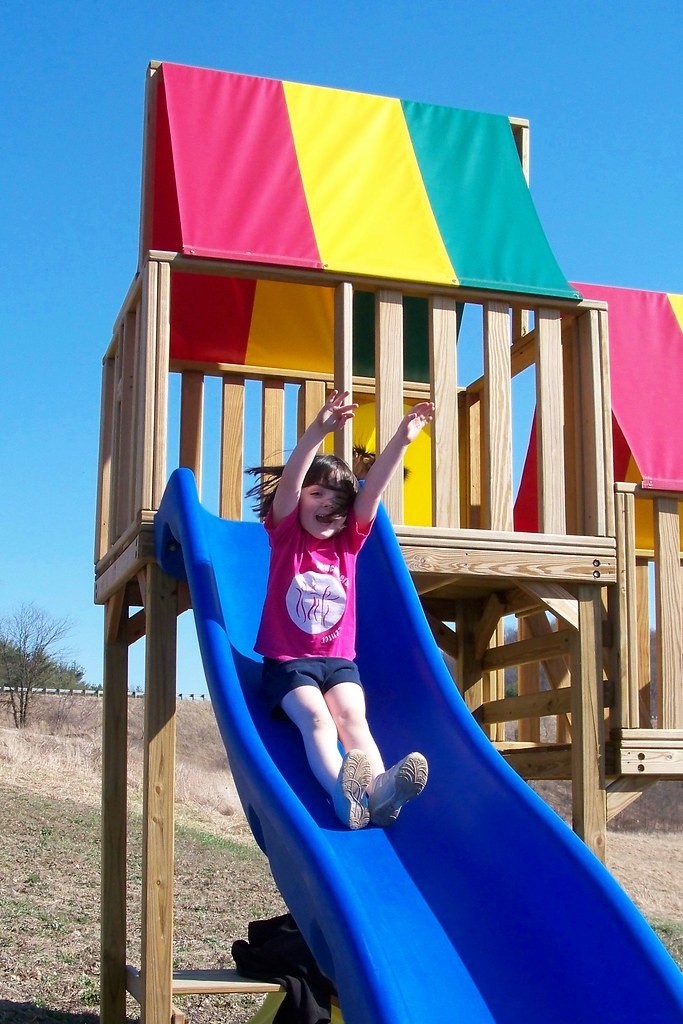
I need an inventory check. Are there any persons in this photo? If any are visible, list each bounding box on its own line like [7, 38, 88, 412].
[243, 390, 435, 830]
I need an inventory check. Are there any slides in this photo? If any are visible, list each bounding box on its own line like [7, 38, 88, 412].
[152, 464, 683, 1024]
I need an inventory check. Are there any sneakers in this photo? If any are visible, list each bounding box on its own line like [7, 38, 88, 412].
[368, 751, 428, 826]
[333, 748, 372, 830]
